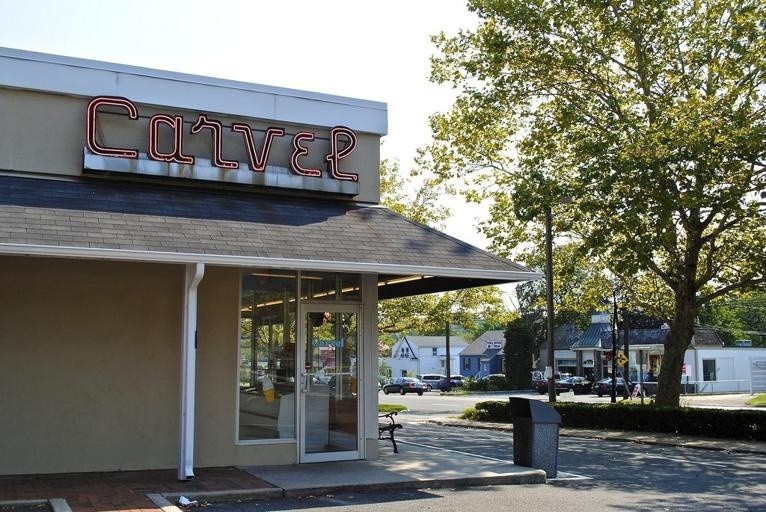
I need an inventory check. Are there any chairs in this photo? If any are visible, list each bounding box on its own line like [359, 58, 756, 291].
[377, 411, 403, 453]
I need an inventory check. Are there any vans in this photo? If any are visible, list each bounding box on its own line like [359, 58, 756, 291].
[414, 373, 456, 394]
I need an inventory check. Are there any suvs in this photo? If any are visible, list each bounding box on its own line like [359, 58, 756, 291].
[327, 374, 382, 394]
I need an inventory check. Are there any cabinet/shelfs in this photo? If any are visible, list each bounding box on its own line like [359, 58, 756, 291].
[240, 384, 329, 452]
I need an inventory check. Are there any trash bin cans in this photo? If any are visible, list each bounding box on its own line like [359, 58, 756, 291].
[510, 397, 563, 479]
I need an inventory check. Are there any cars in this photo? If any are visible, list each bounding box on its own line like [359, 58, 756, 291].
[482, 370, 657, 398]
[381, 376, 427, 397]
[448, 373, 477, 386]
